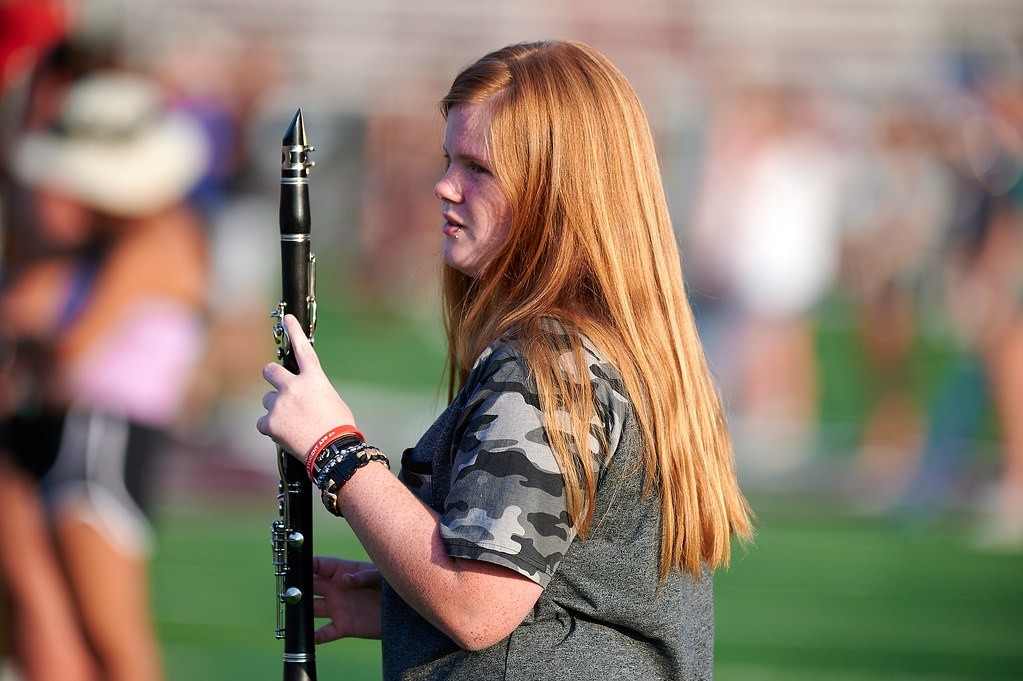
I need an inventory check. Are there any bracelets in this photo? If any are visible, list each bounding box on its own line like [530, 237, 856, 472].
[307, 426, 390, 517]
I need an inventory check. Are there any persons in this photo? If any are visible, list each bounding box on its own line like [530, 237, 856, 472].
[256, 38, 753, 681]
[0, 38, 213, 681]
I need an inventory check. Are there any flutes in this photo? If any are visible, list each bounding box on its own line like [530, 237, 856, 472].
[267, 107, 326, 681]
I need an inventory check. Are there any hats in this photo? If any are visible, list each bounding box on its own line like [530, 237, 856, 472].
[9, 67, 213, 216]
